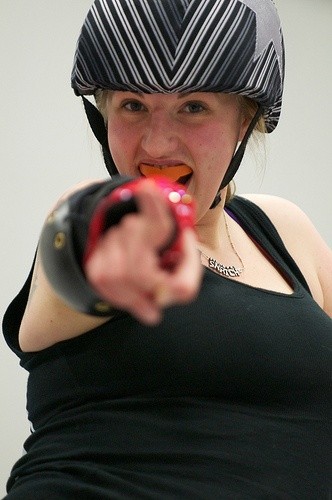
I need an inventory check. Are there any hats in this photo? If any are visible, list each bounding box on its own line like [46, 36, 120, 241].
[71, 0, 286, 133]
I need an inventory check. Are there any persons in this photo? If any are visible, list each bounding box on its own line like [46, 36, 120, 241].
[0, 0, 332, 500]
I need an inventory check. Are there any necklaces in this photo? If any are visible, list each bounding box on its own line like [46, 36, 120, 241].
[193, 210, 245, 277]
